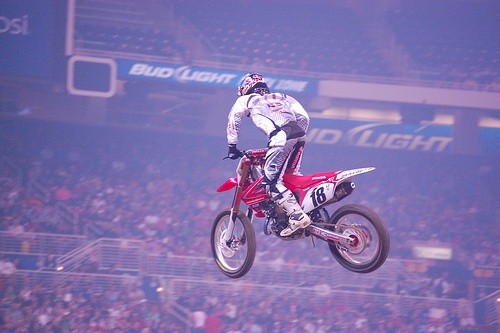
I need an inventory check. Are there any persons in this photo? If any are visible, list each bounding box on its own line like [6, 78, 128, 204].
[226, 71, 313, 236]
[0, 132, 500, 333]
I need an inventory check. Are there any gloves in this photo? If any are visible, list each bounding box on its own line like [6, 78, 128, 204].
[227, 145, 242, 160]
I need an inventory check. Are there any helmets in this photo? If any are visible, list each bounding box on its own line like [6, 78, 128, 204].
[237, 72, 267, 95]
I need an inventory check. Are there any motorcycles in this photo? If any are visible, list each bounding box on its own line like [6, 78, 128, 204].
[210, 140, 390, 279]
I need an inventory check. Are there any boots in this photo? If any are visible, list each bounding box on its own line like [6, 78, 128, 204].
[273, 189, 312, 236]
